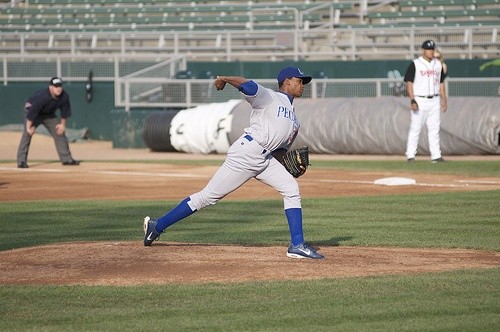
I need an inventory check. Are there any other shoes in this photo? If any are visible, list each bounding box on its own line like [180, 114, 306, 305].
[433, 157, 446, 163]
[18, 163, 29, 168]
[408, 158, 415, 162]
[62, 159, 80, 165]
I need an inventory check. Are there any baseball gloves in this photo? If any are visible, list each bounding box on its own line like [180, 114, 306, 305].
[282, 146, 312, 178]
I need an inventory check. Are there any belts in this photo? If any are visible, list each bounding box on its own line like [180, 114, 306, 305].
[245, 135, 271, 157]
[419, 94, 439, 99]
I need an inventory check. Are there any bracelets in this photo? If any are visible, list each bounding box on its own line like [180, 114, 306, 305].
[411, 99, 416, 103]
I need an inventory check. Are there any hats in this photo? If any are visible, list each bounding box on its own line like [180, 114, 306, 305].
[276, 67, 312, 84]
[49, 78, 65, 86]
[422, 40, 436, 50]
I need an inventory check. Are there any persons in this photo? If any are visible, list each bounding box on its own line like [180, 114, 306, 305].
[434, 49, 446, 77]
[404, 40, 447, 163]
[144, 67, 325, 259]
[17, 77, 80, 168]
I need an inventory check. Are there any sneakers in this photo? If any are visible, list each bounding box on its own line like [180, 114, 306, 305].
[143, 216, 165, 246]
[286, 243, 326, 259]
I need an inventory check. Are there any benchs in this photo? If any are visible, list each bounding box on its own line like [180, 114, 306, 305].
[0, 0, 500, 39]
